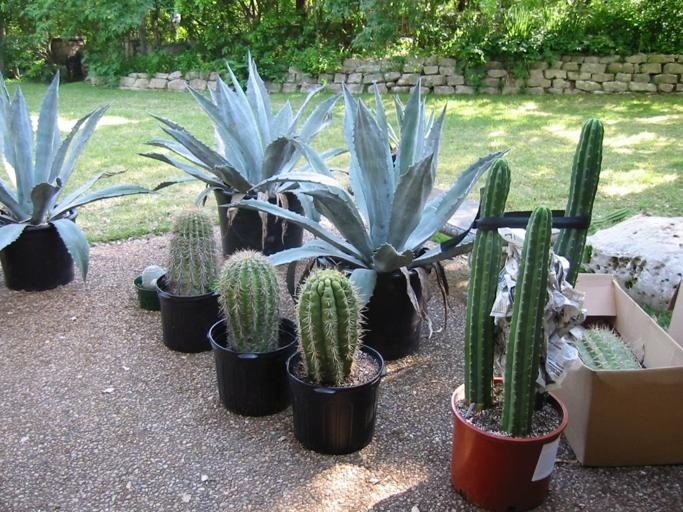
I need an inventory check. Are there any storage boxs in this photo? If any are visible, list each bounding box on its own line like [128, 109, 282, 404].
[541, 272, 682, 469]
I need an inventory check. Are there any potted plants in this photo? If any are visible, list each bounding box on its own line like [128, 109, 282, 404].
[447, 119, 604, 512]
[230, 76, 509, 363]
[133, 49, 349, 265]
[154, 208, 222, 356]
[206, 247, 300, 419]
[0, 73, 150, 296]
[286, 266, 384, 456]
[133, 264, 164, 311]
